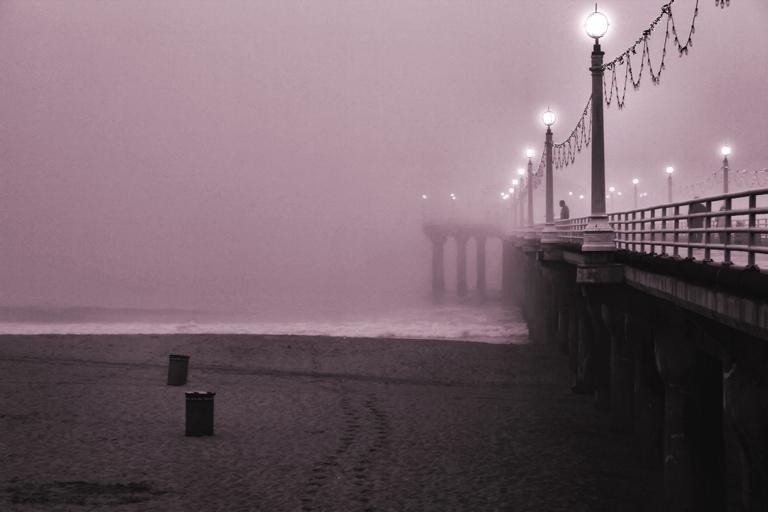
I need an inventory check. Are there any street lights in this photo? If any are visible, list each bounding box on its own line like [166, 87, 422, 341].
[721, 146, 730, 208]
[542, 105, 557, 223]
[666, 167, 673, 221]
[632, 179, 639, 210]
[512, 180, 518, 224]
[519, 168, 524, 225]
[584, 2, 610, 216]
[526, 148, 535, 224]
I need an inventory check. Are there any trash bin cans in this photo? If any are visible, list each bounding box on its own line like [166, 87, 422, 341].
[185, 391, 215, 437]
[168, 355, 190, 386]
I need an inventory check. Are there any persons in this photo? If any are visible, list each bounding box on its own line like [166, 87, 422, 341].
[688, 195, 707, 248]
[715, 205, 726, 244]
[559, 200, 569, 219]
